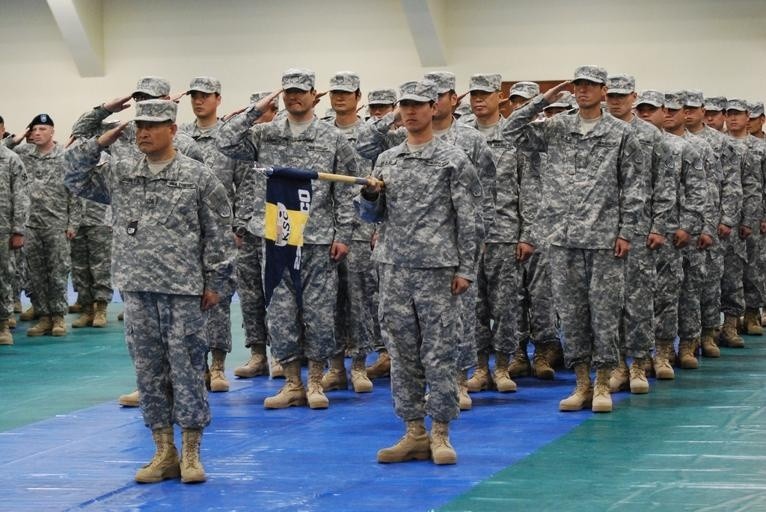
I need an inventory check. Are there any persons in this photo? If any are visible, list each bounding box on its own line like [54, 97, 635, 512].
[71, 79, 203, 406]
[62, 100, 241, 485]
[174, 65, 766, 464]
[1, 114, 124, 344]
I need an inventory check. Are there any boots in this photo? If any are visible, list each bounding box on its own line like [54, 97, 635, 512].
[427, 418, 457, 465]
[119, 388, 138, 407]
[135, 428, 179, 481]
[2, 300, 108, 347]
[378, 419, 428, 462]
[208, 348, 389, 409]
[180, 431, 204, 482]
[452, 340, 560, 412]
[559, 306, 765, 413]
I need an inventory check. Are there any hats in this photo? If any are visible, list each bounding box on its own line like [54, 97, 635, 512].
[605, 73, 636, 94]
[252, 91, 279, 112]
[543, 90, 572, 110]
[368, 89, 397, 107]
[28, 113, 55, 128]
[664, 90, 685, 110]
[508, 81, 541, 100]
[282, 68, 315, 91]
[132, 78, 220, 123]
[704, 97, 727, 112]
[393, 81, 439, 105]
[634, 90, 666, 110]
[324, 108, 337, 118]
[468, 73, 502, 93]
[454, 101, 473, 116]
[684, 90, 705, 107]
[570, 66, 608, 86]
[571, 93, 580, 108]
[746, 101, 765, 118]
[726, 98, 748, 112]
[424, 71, 455, 93]
[329, 71, 360, 92]
